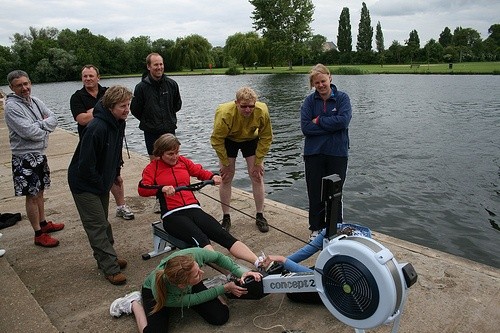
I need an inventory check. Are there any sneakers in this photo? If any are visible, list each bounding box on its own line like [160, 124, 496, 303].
[116, 204, 134, 220]
[110, 290, 144, 318]
[105, 271, 126, 285]
[218, 218, 231, 231]
[256, 215, 269, 232]
[204, 274, 228, 288]
[308, 229, 322, 242]
[118, 260, 127, 268]
[34, 233, 59, 247]
[42, 221, 64, 232]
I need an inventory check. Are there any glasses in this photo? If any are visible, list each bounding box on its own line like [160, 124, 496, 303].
[239, 101, 255, 108]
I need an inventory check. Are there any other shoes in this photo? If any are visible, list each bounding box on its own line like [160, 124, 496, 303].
[0, 250, 5, 256]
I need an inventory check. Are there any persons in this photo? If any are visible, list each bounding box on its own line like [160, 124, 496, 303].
[264, 223, 372, 306]
[70, 65, 134, 220]
[210, 87, 273, 233]
[130, 53, 182, 214]
[301, 64, 352, 243]
[138, 133, 274, 283]
[4, 70, 65, 247]
[0, 233, 5, 257]
[110, 248, 263, 333]
[68, 87, 132, 285]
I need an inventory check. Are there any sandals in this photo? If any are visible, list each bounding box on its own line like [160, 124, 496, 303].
[254, 250, 275, 272]
